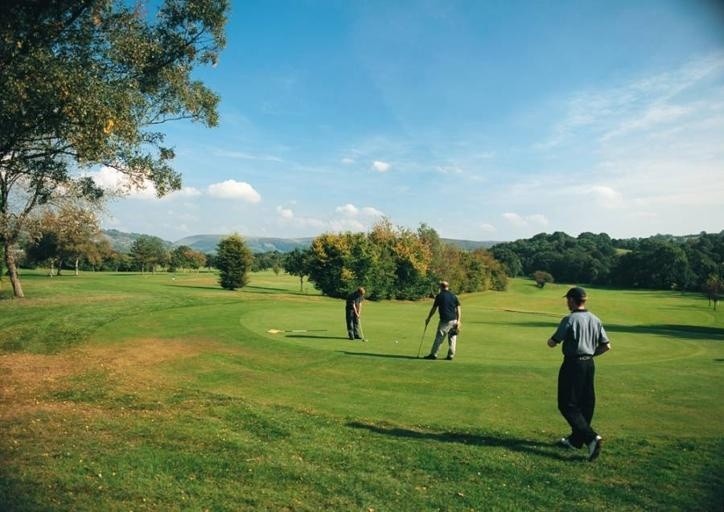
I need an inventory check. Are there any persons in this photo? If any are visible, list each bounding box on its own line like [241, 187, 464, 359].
[424, 282, 460, 360]
[548, 287, 610, 461]
[346, 288, 366, 340]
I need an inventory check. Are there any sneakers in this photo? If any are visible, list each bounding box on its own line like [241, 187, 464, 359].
[424, 354, 452, 360]
[587, 435, 602, 461]
[560, 437, 581, 451]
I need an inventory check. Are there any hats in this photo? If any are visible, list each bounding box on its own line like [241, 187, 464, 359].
[563, 287, 587, 298]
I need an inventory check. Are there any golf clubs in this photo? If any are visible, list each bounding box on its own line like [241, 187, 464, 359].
[417, 322, 428, 358]
[358, 314, 367, 342]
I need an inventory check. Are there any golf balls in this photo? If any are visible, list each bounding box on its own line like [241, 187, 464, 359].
[394, 341, 398, 345]
